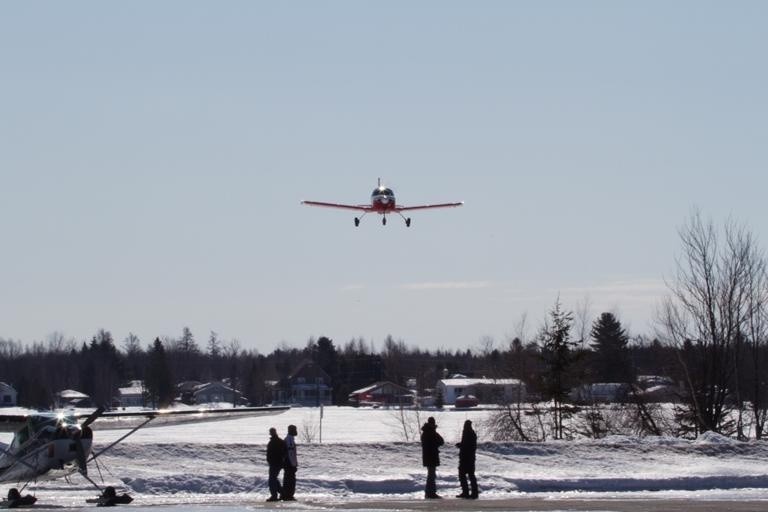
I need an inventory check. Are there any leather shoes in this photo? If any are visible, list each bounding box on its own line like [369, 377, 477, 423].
[456, 493, 478, 500]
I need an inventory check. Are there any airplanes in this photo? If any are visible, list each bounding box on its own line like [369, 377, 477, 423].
[0, 405, 293, 508]
[298, 175, 465, 230]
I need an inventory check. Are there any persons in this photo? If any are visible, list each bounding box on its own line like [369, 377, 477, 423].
[266, 424, 299, 502]
[455, 420, 478, 499]
[420, 417, 445, 499]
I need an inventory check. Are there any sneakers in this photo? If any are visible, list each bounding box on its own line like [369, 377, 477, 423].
[267, 494, 296, 501]
[425, 493, 441, 498]
